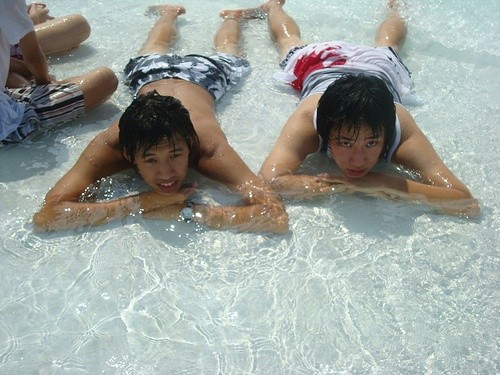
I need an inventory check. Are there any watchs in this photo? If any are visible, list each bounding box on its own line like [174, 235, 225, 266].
[182, 201, 196, 224]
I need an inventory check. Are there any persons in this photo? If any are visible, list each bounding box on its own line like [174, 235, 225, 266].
[257, 0, 479, 217]
[33, 5, 289, 234]
[0, 0, 118, 146]
